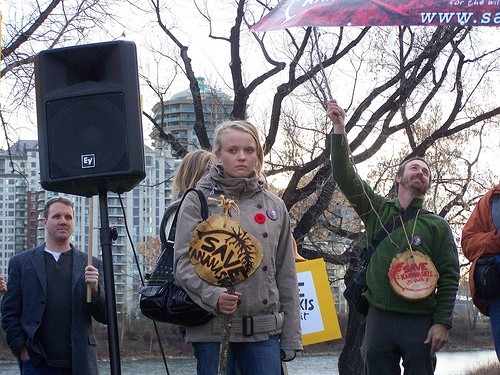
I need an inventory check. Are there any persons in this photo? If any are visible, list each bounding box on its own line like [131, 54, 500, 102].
[161, 151, 217, 250]
[462, 183, 500, 362]
[174, 121, 303, 375]
[327, 99, 460, 375]
[0, 197, 106, 375]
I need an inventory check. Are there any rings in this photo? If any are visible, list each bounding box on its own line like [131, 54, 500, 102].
[89, 276, 90, 278]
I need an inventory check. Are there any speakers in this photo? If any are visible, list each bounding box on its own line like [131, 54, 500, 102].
[36, 40, 146, 198]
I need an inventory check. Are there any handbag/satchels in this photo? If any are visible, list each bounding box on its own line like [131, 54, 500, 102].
[472, 255, 500, 301]
[342, 244, 372, 317]
[138, 187, 219, 327]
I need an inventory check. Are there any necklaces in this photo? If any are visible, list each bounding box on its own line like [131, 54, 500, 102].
[389, 208, 439, 299]
